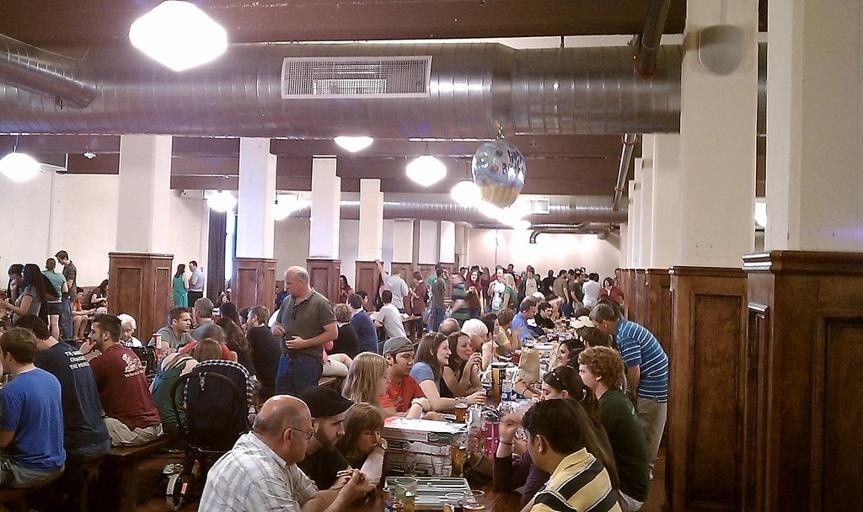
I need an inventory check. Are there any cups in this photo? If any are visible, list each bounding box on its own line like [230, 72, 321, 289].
[565, 319, 570, 328]
[383, 397, 509, 512]
[571, 317, 576, 322]
[501, 379, 512, 400]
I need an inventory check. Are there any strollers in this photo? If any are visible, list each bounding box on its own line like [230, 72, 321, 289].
[167, 360, 256, 508]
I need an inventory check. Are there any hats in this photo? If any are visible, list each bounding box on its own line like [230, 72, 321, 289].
[298, 386, 354, 416]
[383, 336, 415, 352]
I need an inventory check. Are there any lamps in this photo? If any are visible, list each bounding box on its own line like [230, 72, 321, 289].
[129, 1, 229, 73]
[404, 143, 447, 187]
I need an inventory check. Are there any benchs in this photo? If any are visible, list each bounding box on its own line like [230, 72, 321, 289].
[0, 460, 96, 512]
[110, 434, 173, 512]
[318, 376, 345, 391]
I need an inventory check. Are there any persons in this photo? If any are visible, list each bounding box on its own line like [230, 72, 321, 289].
[1, 250, 669, 512]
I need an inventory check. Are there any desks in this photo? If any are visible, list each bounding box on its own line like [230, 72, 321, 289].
[345, 474, 520, 512]
[378, 316, 422, 347]
